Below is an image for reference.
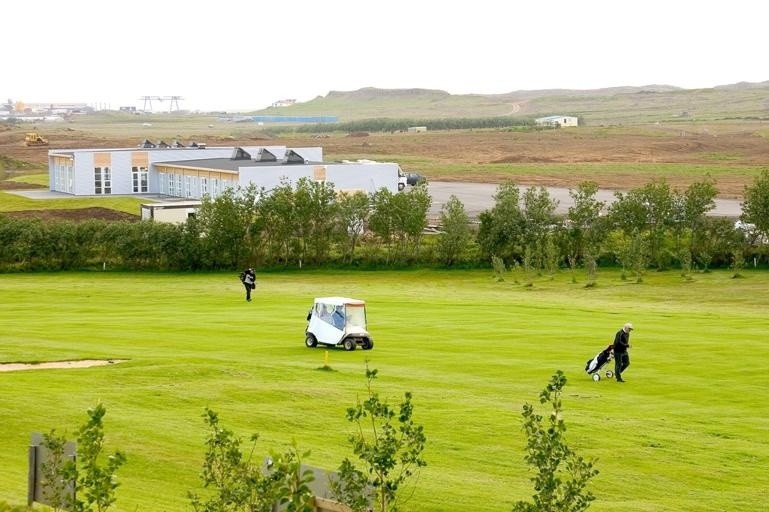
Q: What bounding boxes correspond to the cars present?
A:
[405,172,426,186]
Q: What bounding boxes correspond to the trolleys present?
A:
[585,345,614,382]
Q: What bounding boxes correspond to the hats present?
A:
[252,268,256,272]
[625,324,634,330]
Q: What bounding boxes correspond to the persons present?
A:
[320,305,332,316]
[239,263,256,302]
[613,323,634,383]
[333,305,353,332]
